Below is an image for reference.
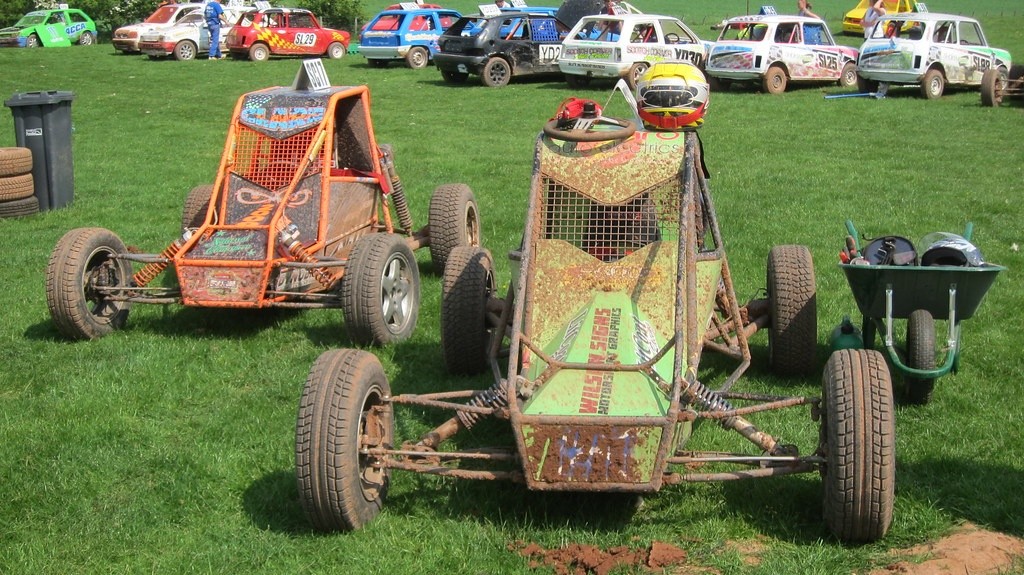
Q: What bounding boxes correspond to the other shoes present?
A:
[218,54,226,60]
[208,56,217,60]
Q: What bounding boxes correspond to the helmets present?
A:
[920,238,986,268]
[635,61,710,133]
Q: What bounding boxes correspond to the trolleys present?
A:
[835,218,1009,397]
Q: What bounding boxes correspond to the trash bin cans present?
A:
[4,88,75,211]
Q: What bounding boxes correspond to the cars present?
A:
[431,3,584,88]
[111,0,279,61]
[556,12,716,93]
[224,0,352,64]
[356,2,476,70]
[704,6,860,96]
[358,0,455,41]
[469,0,621,41]
[855,1,1012,101]
[0,4,99,49]
[841,0,922,35]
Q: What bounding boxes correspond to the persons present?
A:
[598,0,622,34]
[495,0,510,8]
[798,0,820,19]
[860,0,886,41]
[204,0,230,59]
